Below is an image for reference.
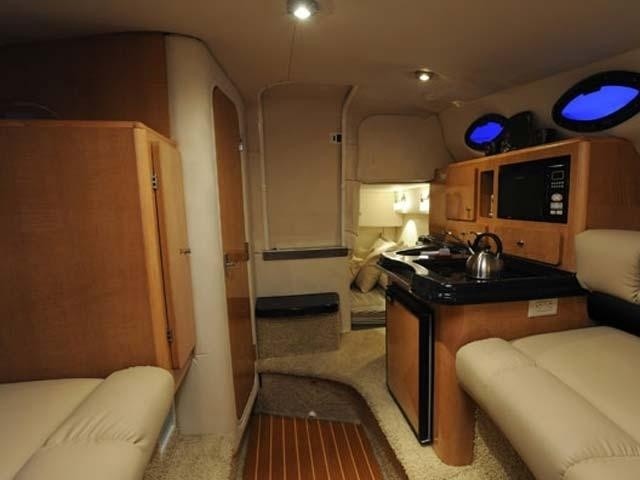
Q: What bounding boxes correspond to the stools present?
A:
[255,291,343,360]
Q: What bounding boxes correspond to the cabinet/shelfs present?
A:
[0,118,198,396]
[384,272,596,467]
[427,135,640,269]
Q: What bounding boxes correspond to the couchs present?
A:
[453,225,640,480]
[1,363,174,479]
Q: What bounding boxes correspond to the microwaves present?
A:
[496,154,572,223]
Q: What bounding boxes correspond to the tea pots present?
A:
[464,233,505,280]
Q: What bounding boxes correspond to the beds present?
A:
[349,280,385,324]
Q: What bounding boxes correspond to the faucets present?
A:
[443,229,463,245]
[468,230,481,245]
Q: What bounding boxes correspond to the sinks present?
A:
[395,244,463,257]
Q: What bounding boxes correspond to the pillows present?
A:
[378,271,389,289]
[355,233,398,294]
[349,256,366,283]
